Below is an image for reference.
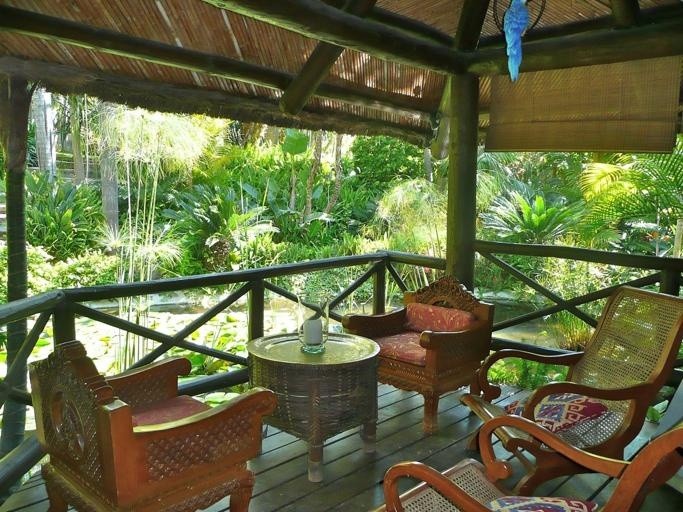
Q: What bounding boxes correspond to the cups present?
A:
[297,292,329,354]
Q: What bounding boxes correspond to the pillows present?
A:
[485,496,598,512]
[406,303,476,332]
[504,393,608,433]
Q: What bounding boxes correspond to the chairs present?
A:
[377,415,683,512]
[342,275,495,434]
[459,285,683,495]
[28,339,279,512]
